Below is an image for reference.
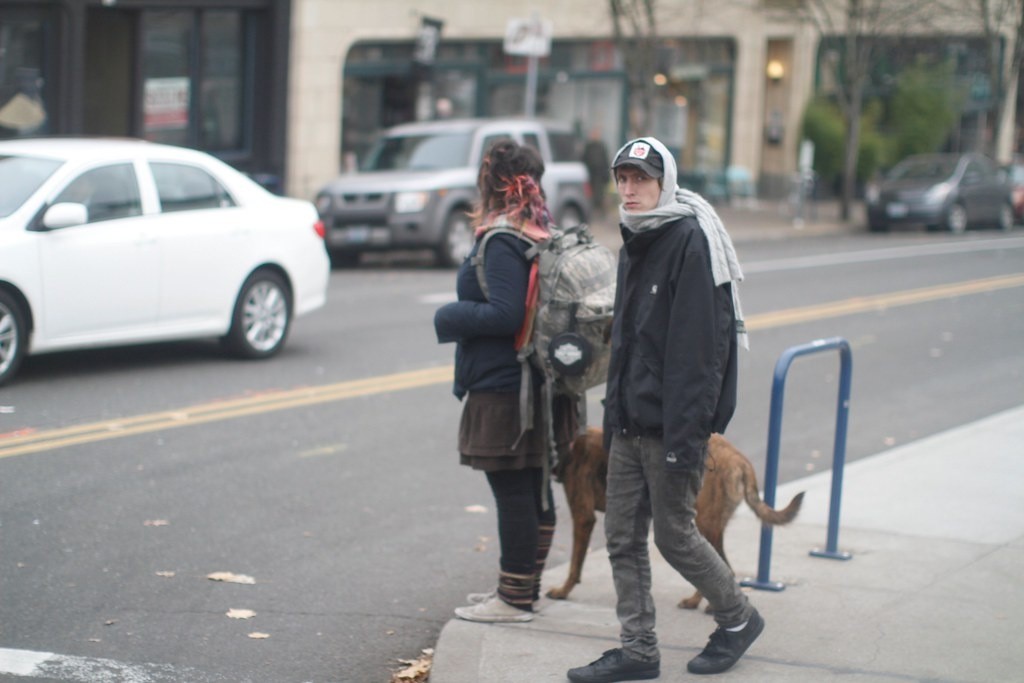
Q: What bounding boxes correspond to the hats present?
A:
[610,139,663,181]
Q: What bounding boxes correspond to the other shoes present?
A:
[455,599,535,621]
[466,590,498,604]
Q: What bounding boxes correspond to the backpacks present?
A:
[474,223,621,396]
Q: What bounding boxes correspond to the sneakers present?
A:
[687,609,764,674]
[566,646,659,682]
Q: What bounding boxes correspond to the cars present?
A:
[1002,160,1024,222]
[866,150,1013,229]
[0,137,330,382]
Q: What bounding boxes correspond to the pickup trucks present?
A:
[316,116,592,269]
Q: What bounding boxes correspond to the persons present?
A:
[558,137,769,682]
[433,139,585,623]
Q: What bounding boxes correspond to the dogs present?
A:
[547,424,806,615]
